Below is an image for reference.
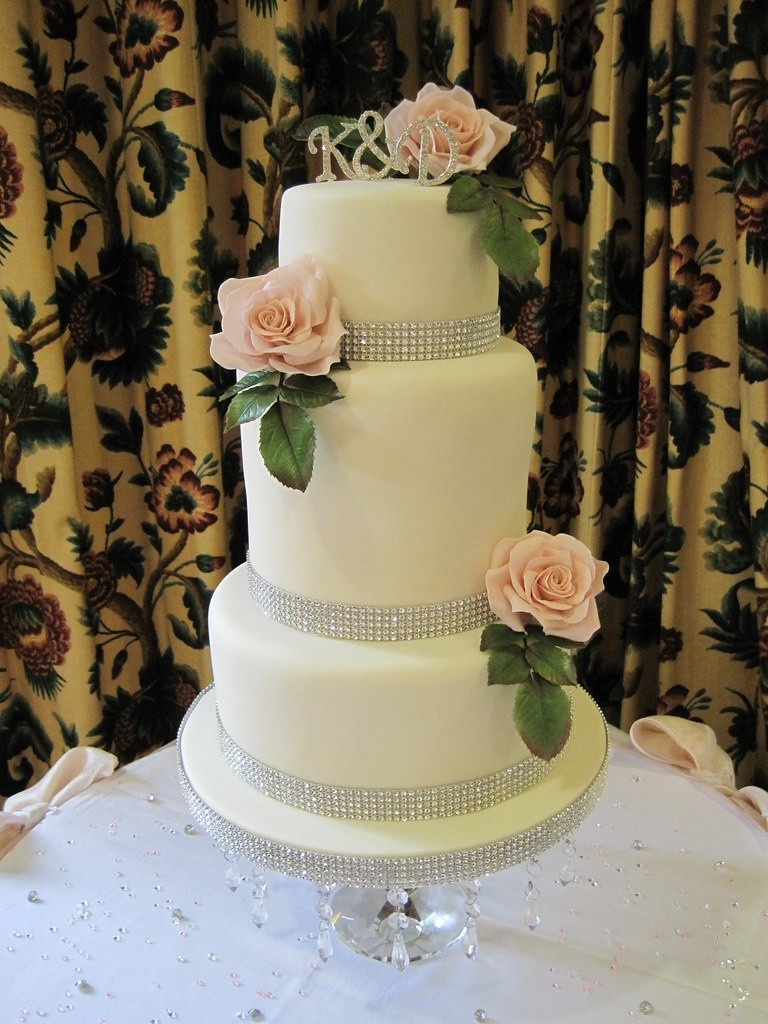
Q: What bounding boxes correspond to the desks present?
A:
[0,723,768,1024]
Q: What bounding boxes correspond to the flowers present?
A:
[201,250,351,496]
[293,81,540,293]
[479,530,610,766]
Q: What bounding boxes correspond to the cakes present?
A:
[174,81,611,887]
